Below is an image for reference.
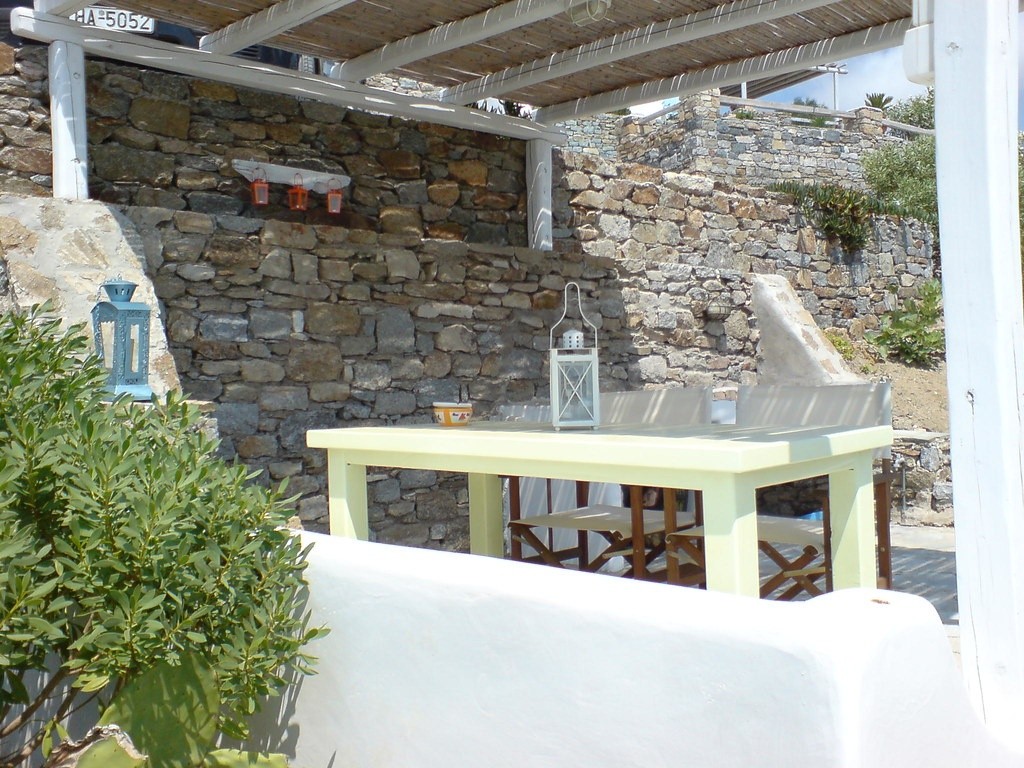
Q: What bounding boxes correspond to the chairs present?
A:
[662,382,895,601]
[497,385,713,588]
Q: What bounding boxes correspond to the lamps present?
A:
[89,272,154,403]
[700,298,733,322]
[546,280,602,431]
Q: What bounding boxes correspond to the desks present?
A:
[307,419,894,598]
